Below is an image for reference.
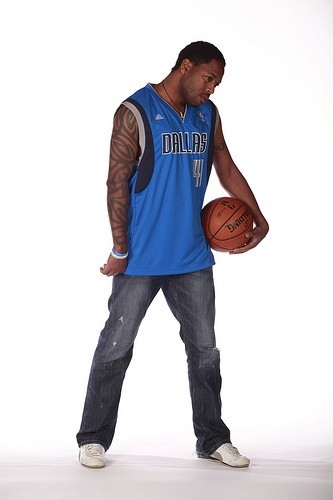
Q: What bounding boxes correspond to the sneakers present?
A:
[211,444,250,468]
[79,443,106,469]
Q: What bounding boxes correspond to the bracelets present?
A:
[111,248,128,259]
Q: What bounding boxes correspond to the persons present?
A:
[76,41,269,468]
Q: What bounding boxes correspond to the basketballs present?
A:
[201,197,253,252]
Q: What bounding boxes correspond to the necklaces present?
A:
[162,81,186,120]
